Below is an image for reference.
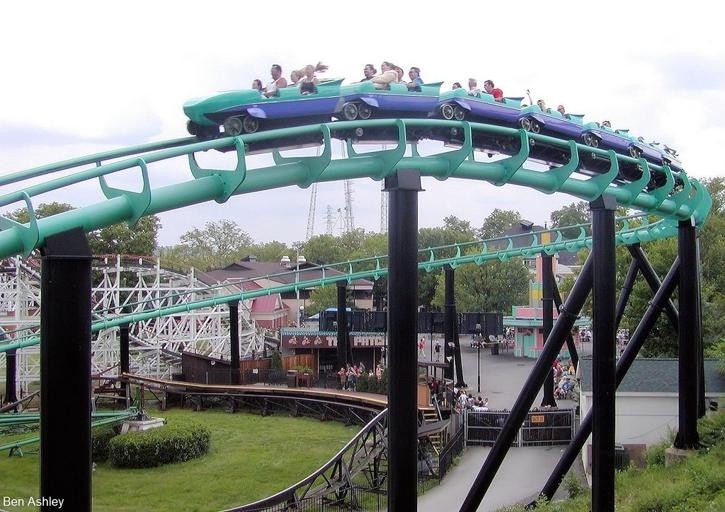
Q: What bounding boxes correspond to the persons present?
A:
[299,297,629,409]
[252,61,610,128]
[709,402,718,411]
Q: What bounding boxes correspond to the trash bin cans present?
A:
[287,370,298,387]
[491,343,498,355]
[615,444,629,470]
